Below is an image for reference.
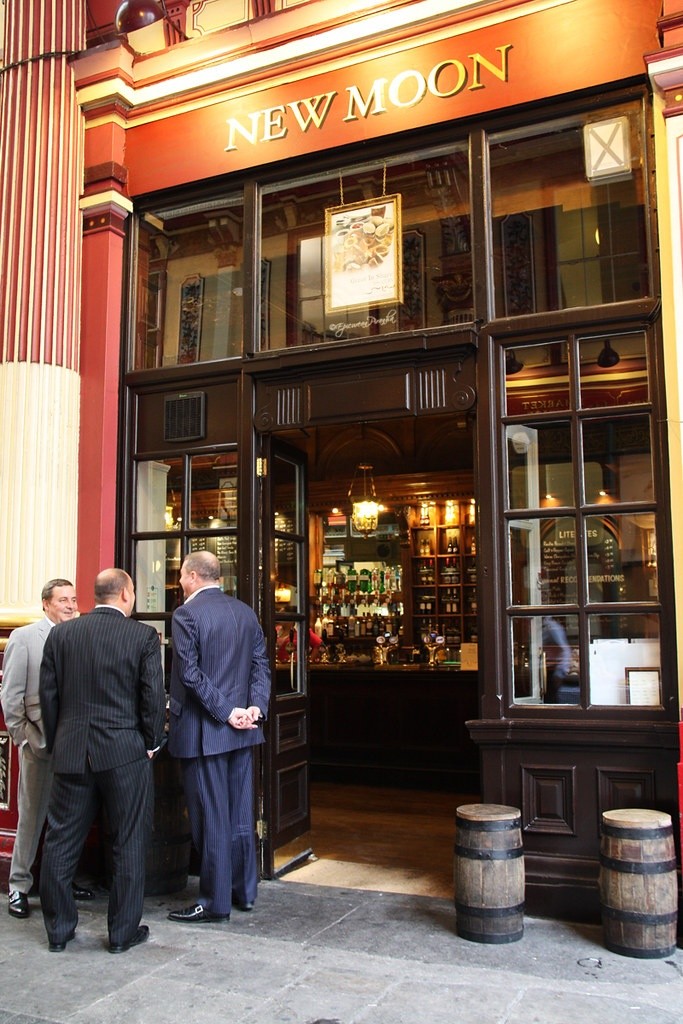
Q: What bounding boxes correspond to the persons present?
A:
[38,569,167,954]
[1,578,77,919]
[275,605,323,662]
[168,551,271,921]
[543,615,572,704]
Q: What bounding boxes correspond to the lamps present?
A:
[114,0,189,41]
[506,350,524,375]
[347,464,388,531]
[597,340,620,368]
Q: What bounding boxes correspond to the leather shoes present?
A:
[48,932,75,952]
[71,883,95,901]
[109,925,148,953]
[167,904,230,922]
[8,892,30,918]
[232,888,254,911]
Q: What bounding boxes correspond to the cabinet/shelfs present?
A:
[277,493,478,650]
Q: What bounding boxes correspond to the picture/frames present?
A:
[261,258,270,350]
[176,273,204,365]
[324,193,403,316]
[500,212,537,316]
[395,229,427,332]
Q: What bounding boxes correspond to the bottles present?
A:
[446,588,458,613]
[419,538,431,556]
[314,565,402,595]
[445,618,460,644]
[471,588,477,614]
[420,508,430,526]
[420,559,434,585]
[470,558,477,583]
[314,612,406,636]
[470,616,478,643]
[316,595,404,616]
[419,589,432,614]
[420,618,433,643]
[471,536,476,554]
[442,557,459,583]
[447,536,458,554]
